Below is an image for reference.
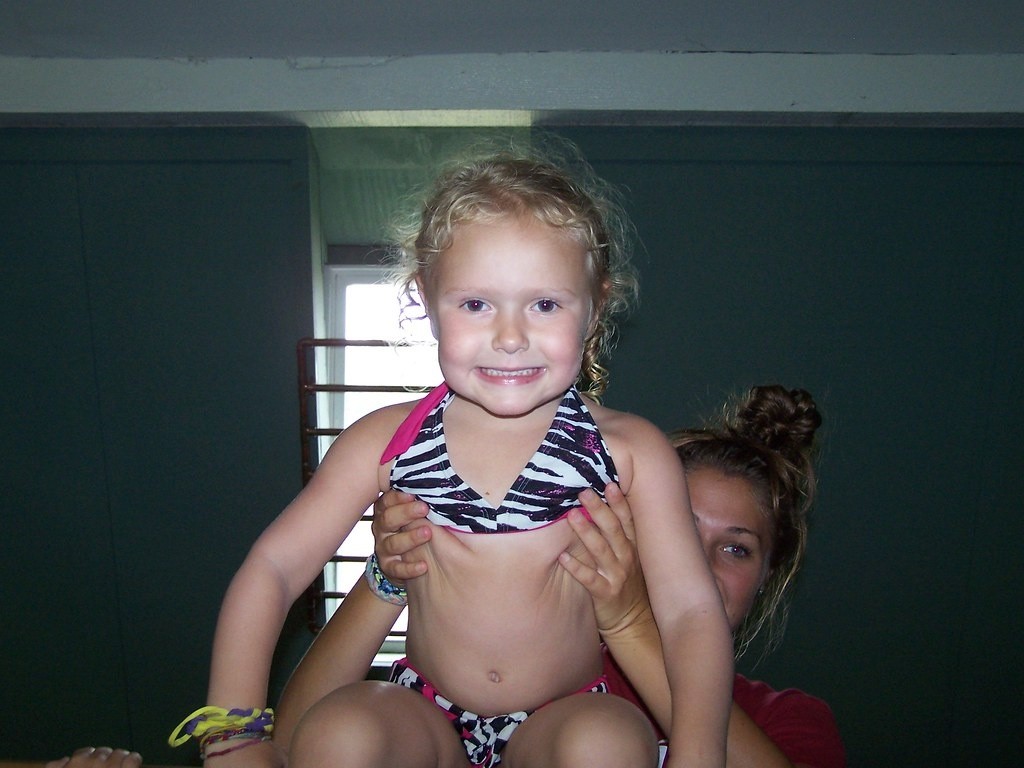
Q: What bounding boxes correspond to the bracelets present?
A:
[365,553,407,597]
[168,706,276,760]
[365,553,408,606]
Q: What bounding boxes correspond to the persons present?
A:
[272,385,847,768]
[201,150,732,768]
